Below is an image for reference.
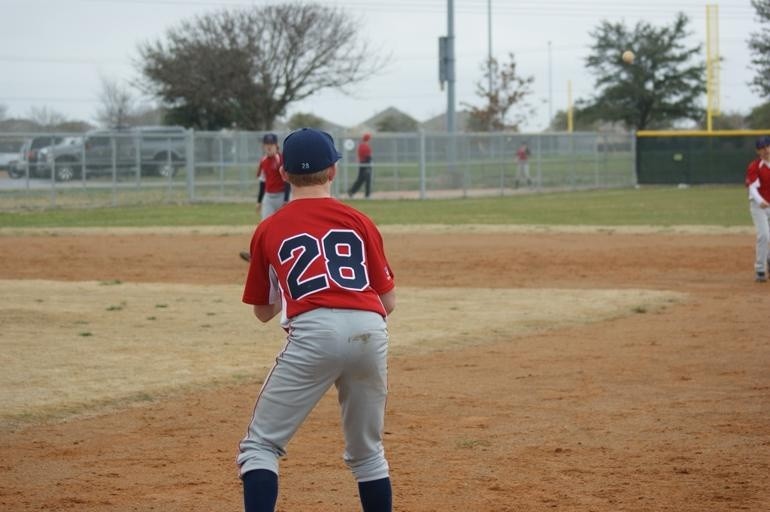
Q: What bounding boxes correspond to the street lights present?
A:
[623,51,646,130]
[547,40,553,123]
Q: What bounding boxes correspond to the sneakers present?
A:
[238,250,251,263]
[755,263,770,284]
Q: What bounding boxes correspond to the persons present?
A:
[236,128,396,511]
[347,133,372,199]
[515,143,533,188]
[744,136,770,282]
[256,133,291,221]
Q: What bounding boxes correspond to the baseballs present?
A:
[623,51,636,62]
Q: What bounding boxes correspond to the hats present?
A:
[282,127,343,177]
[262,133,279,146]
[755,136,770,149]
[362,132,372,142]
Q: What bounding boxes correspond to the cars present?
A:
[0,124,192,182]
